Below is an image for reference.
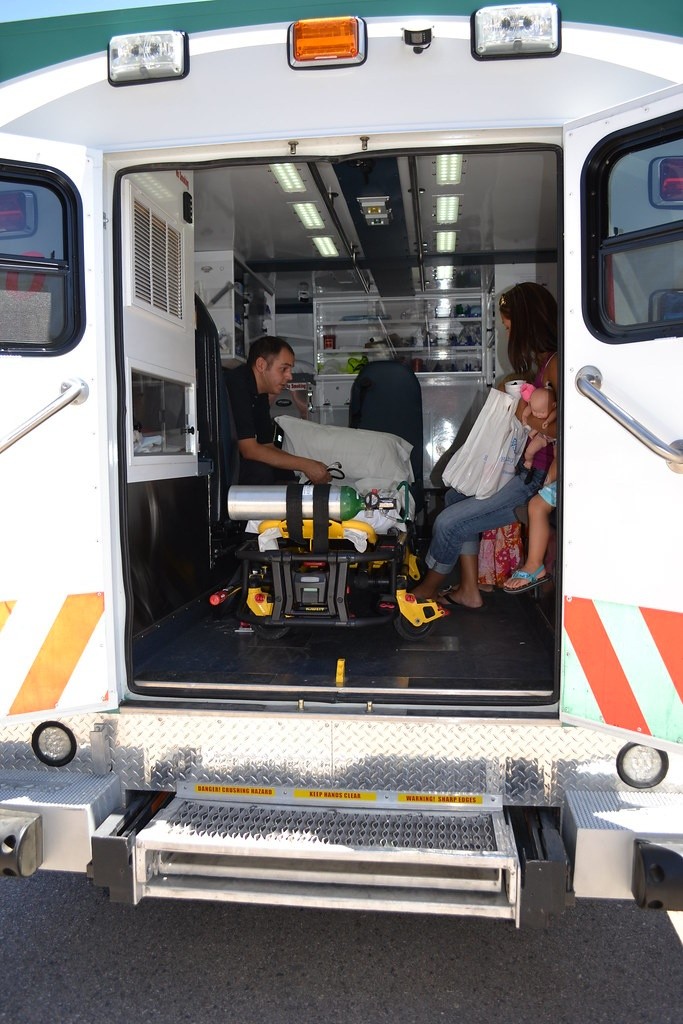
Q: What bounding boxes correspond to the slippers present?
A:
[436,591,489,614]
[478,583,495,594]
[438,585,457,597]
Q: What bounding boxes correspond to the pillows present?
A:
[275,415,415,485]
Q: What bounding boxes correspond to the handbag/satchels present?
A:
[441,387,529,501]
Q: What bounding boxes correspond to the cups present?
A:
[505,379,526,399]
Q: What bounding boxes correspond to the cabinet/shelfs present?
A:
[312,296,492,381]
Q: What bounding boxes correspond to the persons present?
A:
[220,335,333,487]
[521,388,558,469]
[502,460,556,594]
[410,282,558,610]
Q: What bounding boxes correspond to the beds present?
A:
[241,475,415,638]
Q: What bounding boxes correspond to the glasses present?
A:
[498,297,509,307]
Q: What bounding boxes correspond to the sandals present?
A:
[503,564,553,594]
